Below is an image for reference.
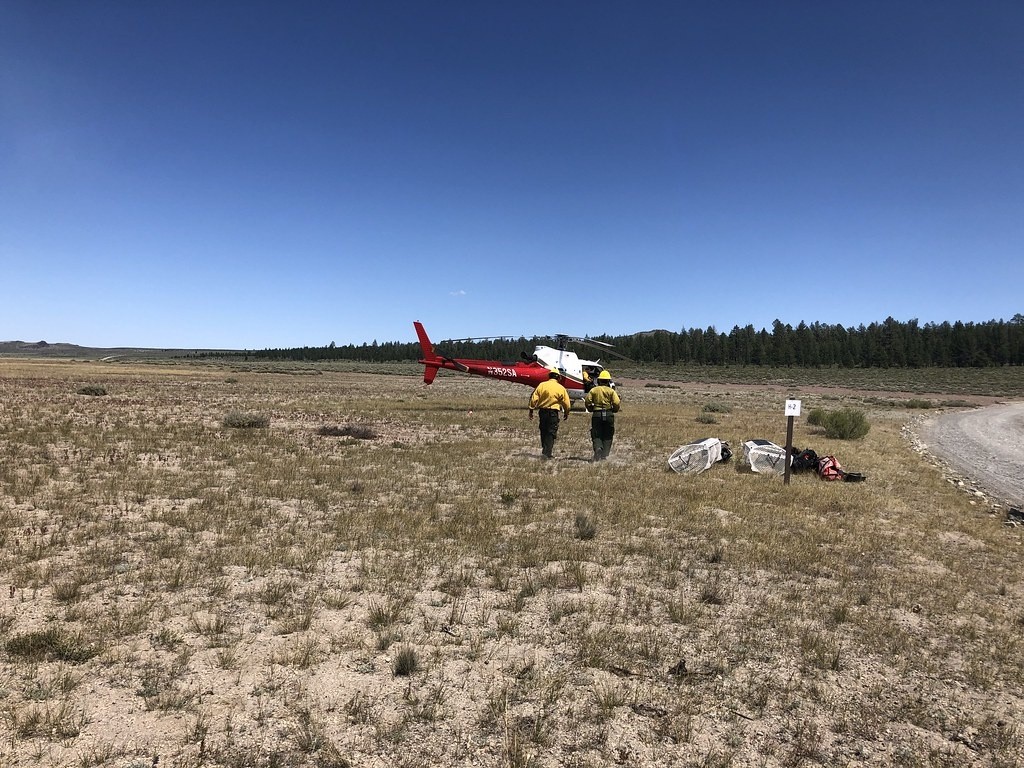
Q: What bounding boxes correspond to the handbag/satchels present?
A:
[781,446,866,481]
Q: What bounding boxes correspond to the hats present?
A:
[585,367,591,373]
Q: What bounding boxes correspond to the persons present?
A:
[528,369,571,458]
[583,368,593,386]
[585,370,620,459]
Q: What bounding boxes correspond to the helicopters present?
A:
[412,320,617,414]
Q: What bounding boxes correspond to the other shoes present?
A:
[595,448,602,460]
[601,456,607,460]
[542,452,555,459]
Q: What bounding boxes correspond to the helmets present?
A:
[548,369,561,376]
[598,370,612,380]
